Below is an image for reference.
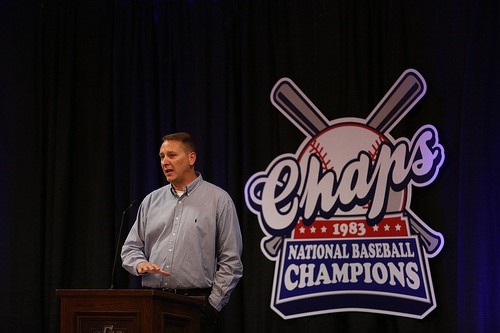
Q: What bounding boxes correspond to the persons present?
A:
[120,130,244,332]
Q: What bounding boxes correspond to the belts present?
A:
[156,285,214,297]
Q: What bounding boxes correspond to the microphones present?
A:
[110,201,134,287]
